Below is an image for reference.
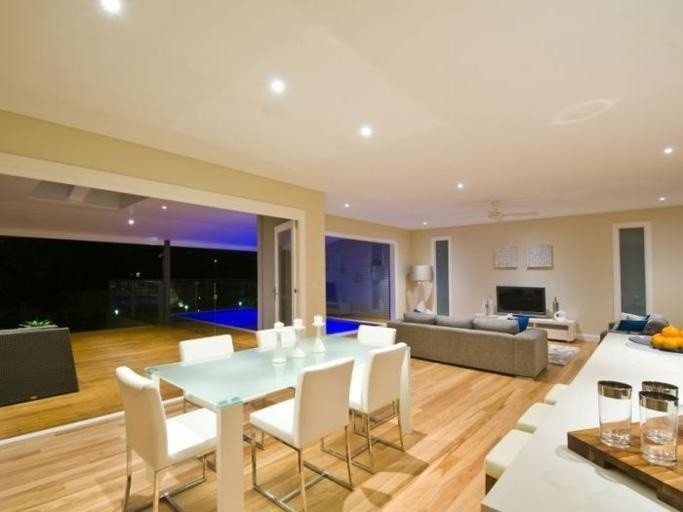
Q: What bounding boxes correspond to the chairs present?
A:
[254,325,298,346]
[320,340,410,477]
[355,323,396,348]
[595,311,671,345]
[114,366,221,512]
[178,331,267,474]
[247,356,355,512]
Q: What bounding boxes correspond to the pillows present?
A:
[616,319,648,332]
[507,313,529,333]
[610,311,649,330]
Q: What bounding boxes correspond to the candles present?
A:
[313,315,322,325]
[294,318,303,328]
[274,320,285,329]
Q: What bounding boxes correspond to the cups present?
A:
[642,377,679,403]
[597,378,633,449]
[638,391,679,467]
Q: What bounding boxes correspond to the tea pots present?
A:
[554,310,568,322]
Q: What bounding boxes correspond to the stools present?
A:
[515,400,555,431]
[540,379,571,406]
[475,425,533,493]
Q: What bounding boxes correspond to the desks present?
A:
[136,329,413,512]
[470,327,682,511]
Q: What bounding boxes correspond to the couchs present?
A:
[385,310,550,379]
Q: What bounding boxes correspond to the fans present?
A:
[486,195,538,220]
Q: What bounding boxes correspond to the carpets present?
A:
[546,341,580,367]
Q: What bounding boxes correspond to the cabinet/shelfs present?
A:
[473,312,578,344]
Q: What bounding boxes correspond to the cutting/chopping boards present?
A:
[566,413,683,510]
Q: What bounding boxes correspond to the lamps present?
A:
[407,262,433,313]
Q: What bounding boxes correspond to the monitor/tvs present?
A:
[496,286,546,320]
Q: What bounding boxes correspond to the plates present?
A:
[629,335,650,347]
